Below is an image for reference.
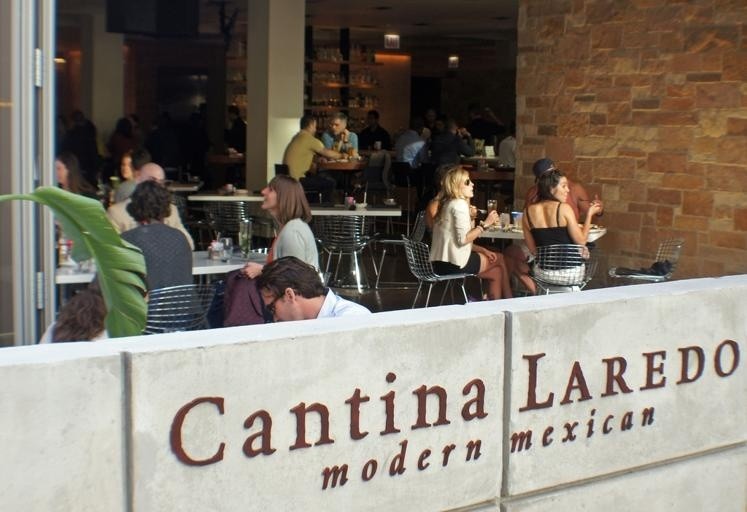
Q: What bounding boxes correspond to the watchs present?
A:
[342,154,344,158]
[344,142,349,143]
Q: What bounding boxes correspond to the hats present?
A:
[533,158,553,183]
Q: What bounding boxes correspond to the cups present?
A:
[220,238,233,263]
[238,220,252,259]
[512,212,523,227]
[487,199,498,216]
[500,213,510,232]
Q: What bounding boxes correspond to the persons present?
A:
[431,122,475,186]
[225,105,247,153]
[107,162,195,250]
[498,126,515,211]
[105,118,142,181]
[421,165,477,252]
[388,119,432,213]
[284,116,348,207]
[521,159,604,222]
[224,174,319,327]
[57,113,83,161]
[505,169,602,295]
[56,150,95,197]
[89,180,202,333]
[136,111,182,181]
[319,113,362,202]
[121,148,136,180]
[424,108,437,130]
[71,109,99,191]
[116,151,151,203]
[430,115,448,138]
[128,113,149,157]
[484,105,507,135]
[358,110,391,150]
[429,167,513,300]
[256,257,372,322]
[466,105,505,150]
[39,270,149,343]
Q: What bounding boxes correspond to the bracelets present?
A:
[477,220,488,232]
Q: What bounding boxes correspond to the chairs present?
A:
[202,200,254,240]
[375,210,427,290]
[312,215,379,295]
[273,163,323,208]
[401,232,484,309]
[143,284,217,336]
[386,161,417,238]
[528,243,599,295]
[609,237,688,282]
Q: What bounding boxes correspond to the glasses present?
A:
[551,169,560,184]
[328,123,340,127]
[464,178,470,186]
[266,288,299,314]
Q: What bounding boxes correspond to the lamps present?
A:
[54,51,68,67]
